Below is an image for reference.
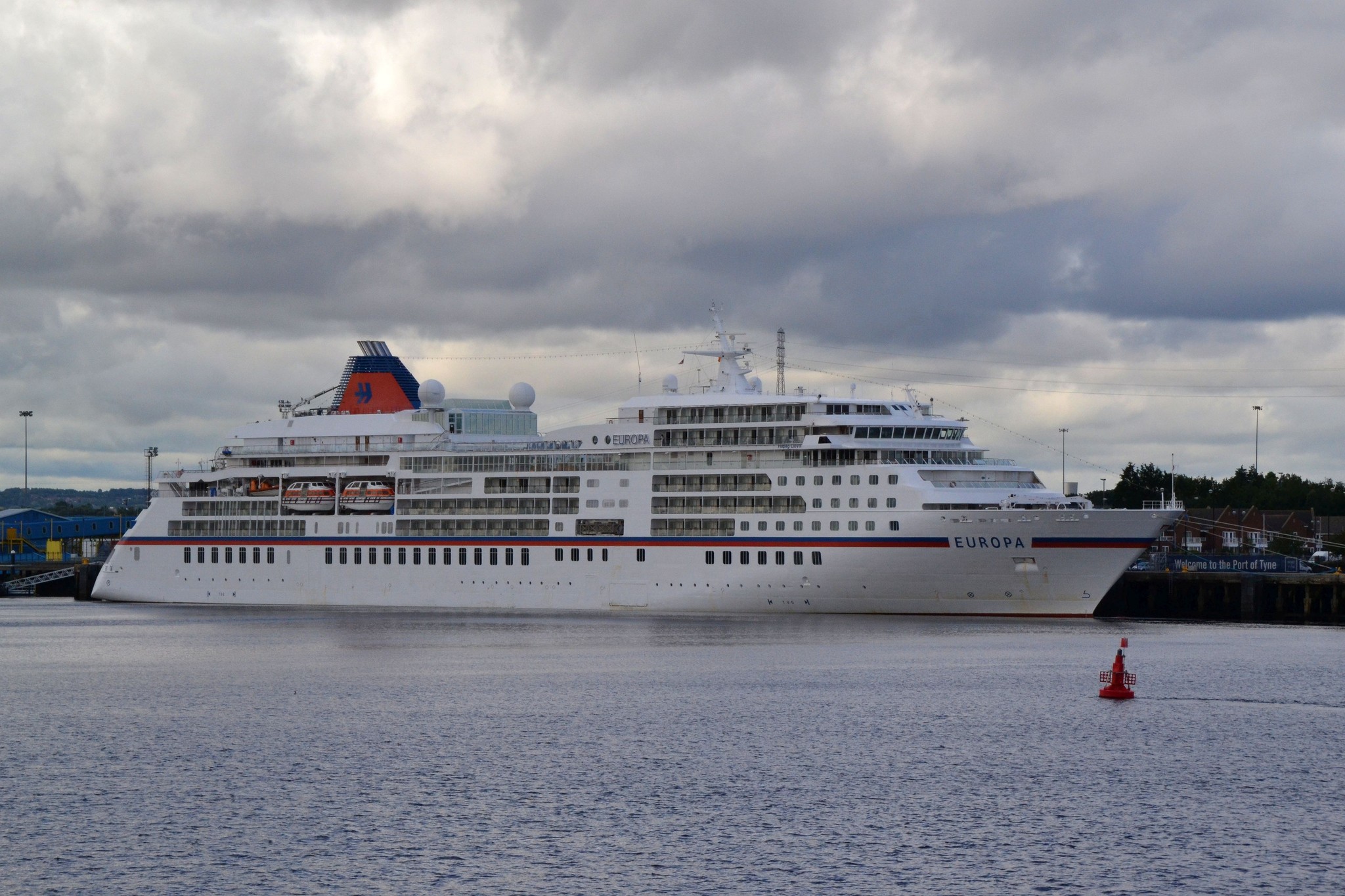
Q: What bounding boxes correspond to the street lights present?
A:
[1252,406,1263,471]
[19,410,33,496]
[148,446,159,507]
[1194,489,1215,555]
[1311,516,1320,552]
[1100,478,1106,509]
[1059,429,1069,495]
[1232,510,1246,555]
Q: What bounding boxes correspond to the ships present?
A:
[88,298,1192,628]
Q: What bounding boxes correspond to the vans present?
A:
[1308,551,1343,563]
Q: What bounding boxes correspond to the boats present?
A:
[250,488,287,496]
[282,481,335,512]
[340,480,395,511]
